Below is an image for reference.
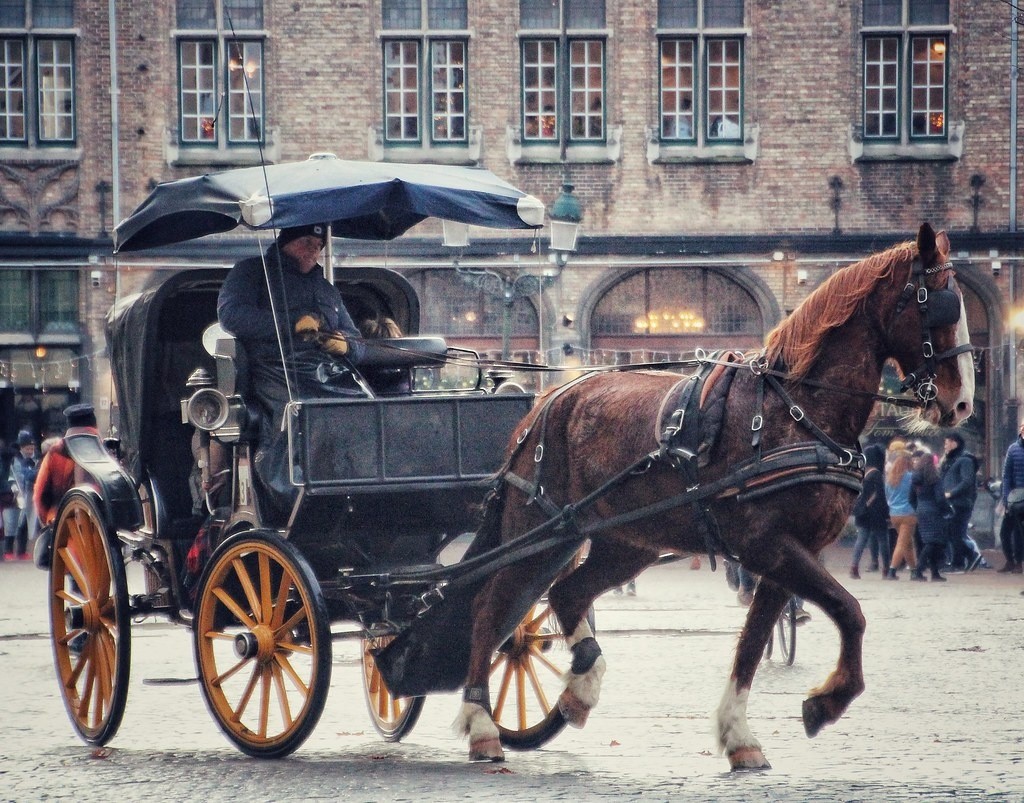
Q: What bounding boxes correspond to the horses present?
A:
[450,219,977,770]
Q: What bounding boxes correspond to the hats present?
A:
[278,224,327,251]
[10,431,35,445]
[62,403,97,427]
[968,523,974,529]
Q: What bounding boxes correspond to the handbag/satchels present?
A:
[1007,488,1024,513]
[16,493,26,509]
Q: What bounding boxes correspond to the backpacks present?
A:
[852,466,880,517]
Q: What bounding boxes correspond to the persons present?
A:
[722,419,1024,620]
[613,580,637,596]
[0,404,102,600]
[217,220,371,452]
[357,316,411,396]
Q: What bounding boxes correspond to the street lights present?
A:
[440,182,584,361]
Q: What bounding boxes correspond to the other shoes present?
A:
[888,568,897,578]
[2,554,15,561]
[931,569,946,582]
[850,564,861,579]
[1012,563,1022,573]
[882,569,899,580]
[16,553,33,561]
[983,562,993,568]
[911,568,917,579]
[866,563,879,572]
[966,552,983,572]
[915,563,927,581]
[997,560,1015,572]
[941,566,965,574]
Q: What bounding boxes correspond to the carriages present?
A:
[33,153,977,773]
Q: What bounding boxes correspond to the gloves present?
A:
[296,316,319,332]
[322,331,349,356]
